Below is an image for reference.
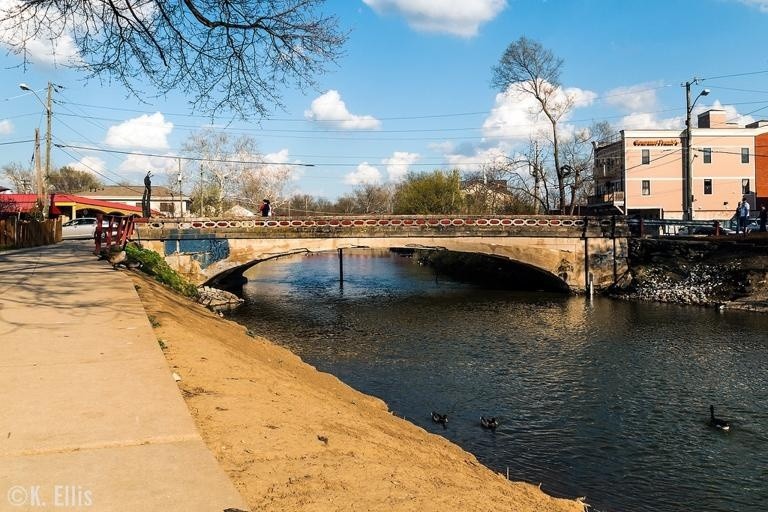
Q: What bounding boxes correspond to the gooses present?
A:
[708,404,731,430]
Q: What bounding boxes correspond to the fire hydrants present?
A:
[713,220,720,237]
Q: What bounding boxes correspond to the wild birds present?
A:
[97,250,127,269]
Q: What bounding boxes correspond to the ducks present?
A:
[123,260,144,271]
[431,411,448,424]
[480,415,499,428]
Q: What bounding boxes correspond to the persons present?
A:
[257,199,269,225]
[736,198,766,235]
[267,200,272,225]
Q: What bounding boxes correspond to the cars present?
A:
[679,225,734,236]
[61,216,120,240]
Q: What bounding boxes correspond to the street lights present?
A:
[683,86,711,220]
[19,81,53,218]
